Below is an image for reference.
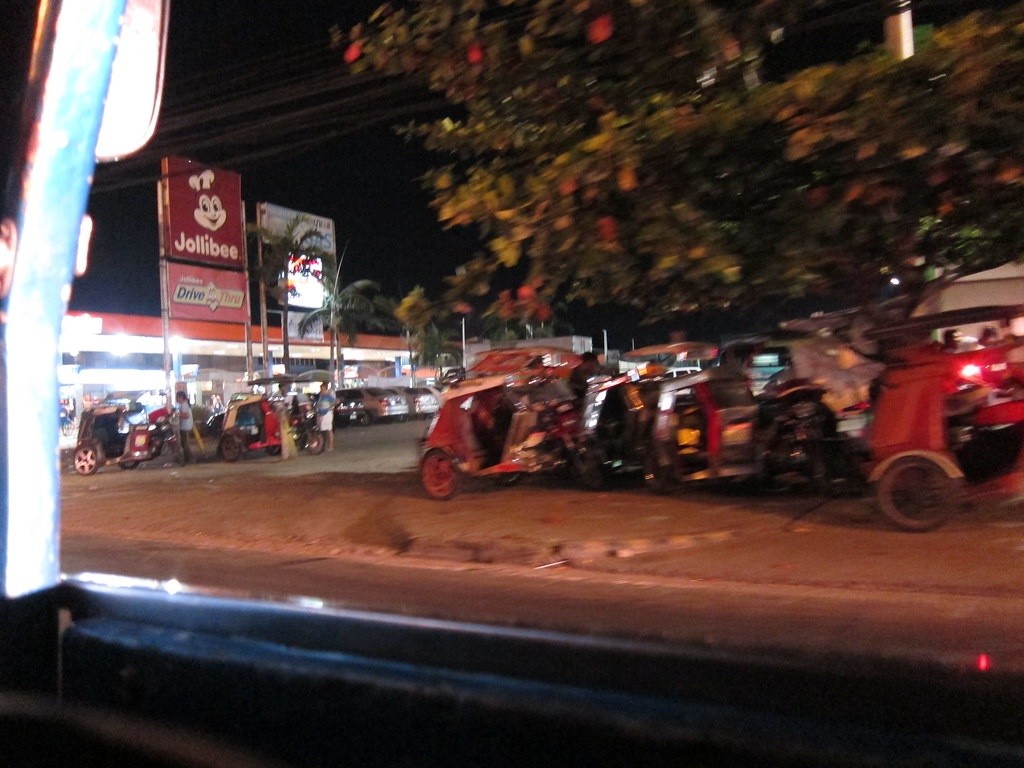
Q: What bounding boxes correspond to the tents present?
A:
[780,261,1024,380]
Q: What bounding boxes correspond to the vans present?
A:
[717,335,793,406]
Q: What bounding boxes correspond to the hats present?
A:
[581,352,596,360]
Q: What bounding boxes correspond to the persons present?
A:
[176,391,197,466]
[981,324,1004,343]
[943,329,965,353]
[317,383,339,450]
[472,385,520,470]
[568,351,611,407]
[58,403,68,436]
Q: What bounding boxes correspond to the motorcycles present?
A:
[72,392,186,477]
[217,394,326,461]
[579,344,761,496]
[752,304,1024,532]
[417,346,585,498]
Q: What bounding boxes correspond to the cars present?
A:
[398,386,440,422]
[358,387,409,427]
[206,392,316,433]
[311,392,366,428]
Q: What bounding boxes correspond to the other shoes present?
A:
[326,447,334,452]
